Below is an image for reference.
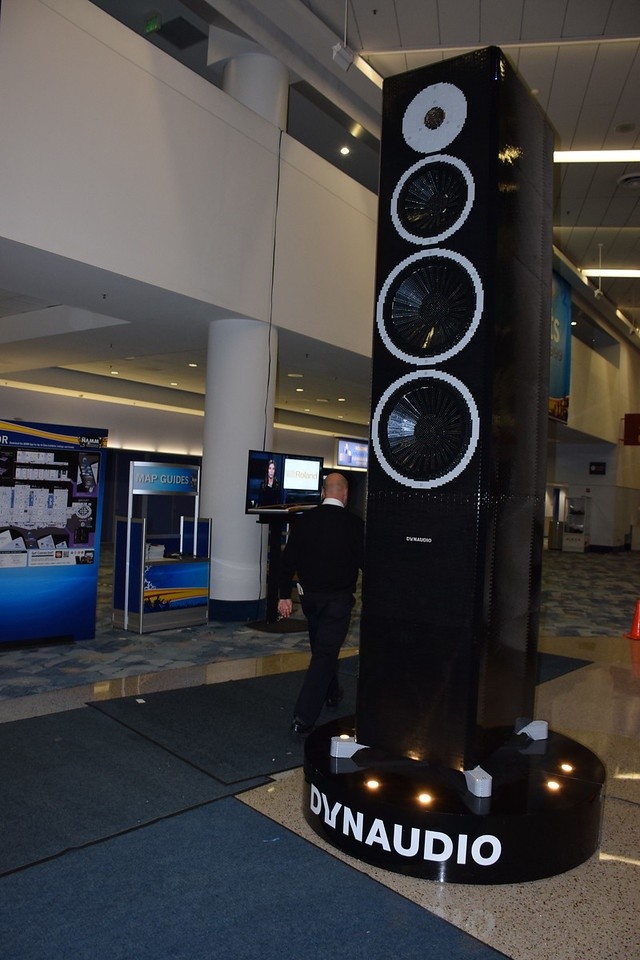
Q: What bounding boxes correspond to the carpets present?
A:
[0,651,596,960]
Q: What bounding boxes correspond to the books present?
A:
[145,543,165,560]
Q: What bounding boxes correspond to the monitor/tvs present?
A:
[338,441,368,469]
[243,449,325,515]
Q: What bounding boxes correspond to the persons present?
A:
[277,473,365,732]
[257,460,280,506]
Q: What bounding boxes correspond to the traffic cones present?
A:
[623,600,640,639]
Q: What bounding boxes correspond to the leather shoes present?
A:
[326,693,344,711]
[290,723,317,739]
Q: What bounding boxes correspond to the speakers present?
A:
[356,45,559,764]
